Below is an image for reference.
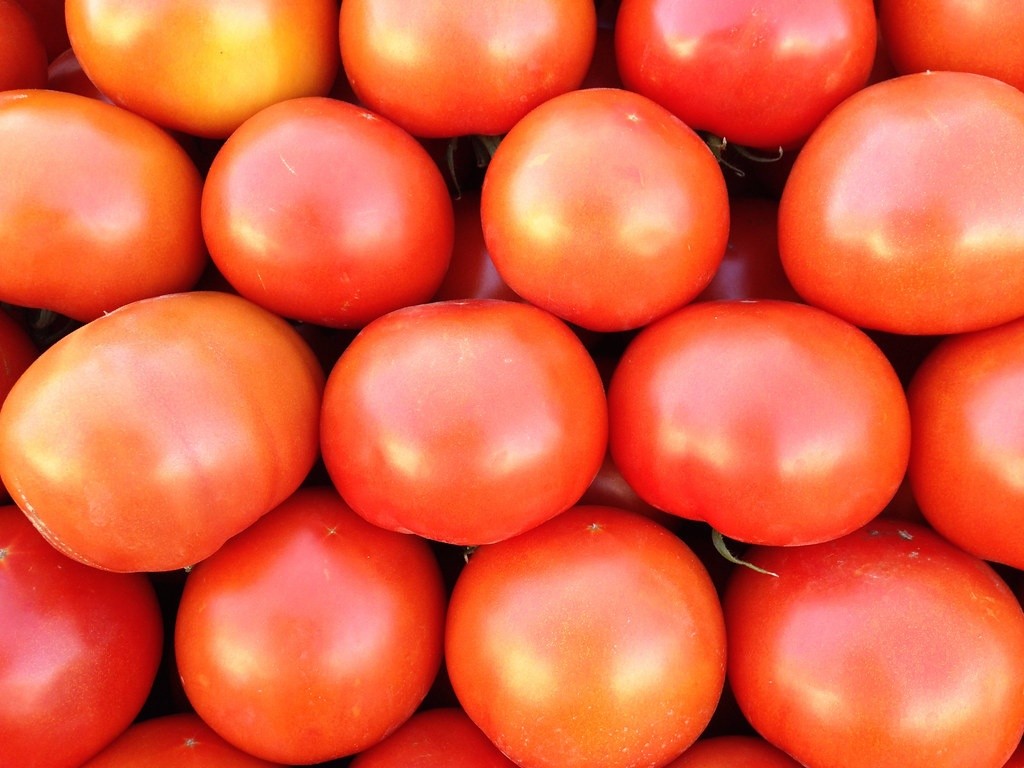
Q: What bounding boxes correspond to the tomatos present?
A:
[0,0,1024,768]
